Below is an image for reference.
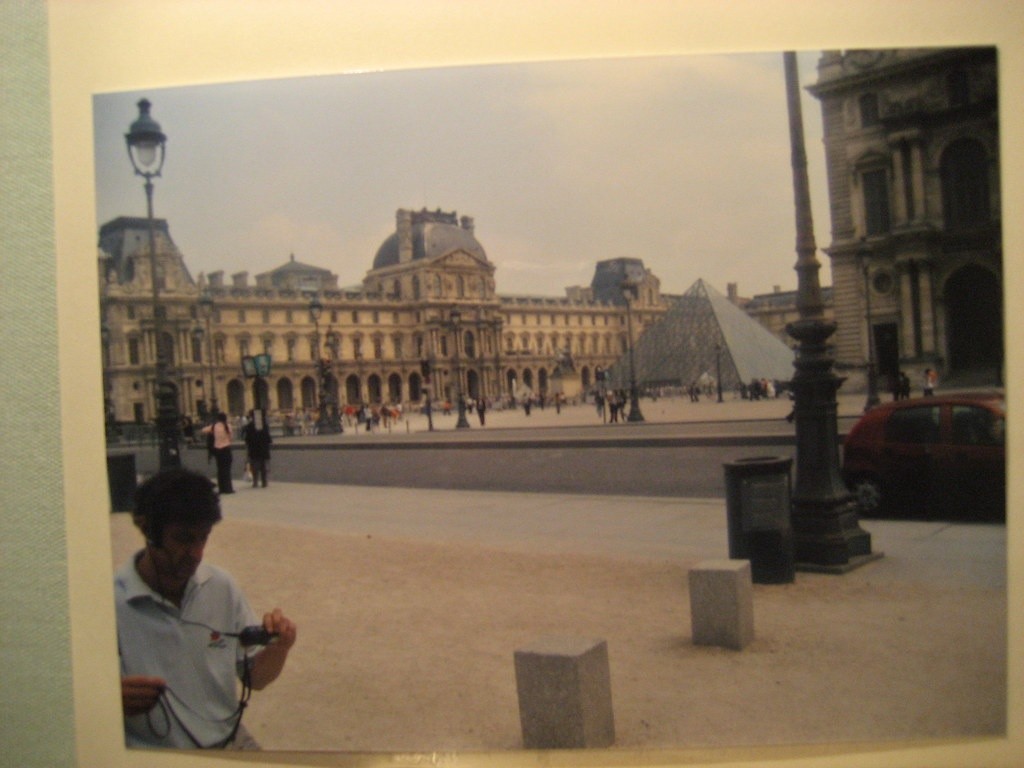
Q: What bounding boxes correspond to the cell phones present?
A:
[240,625,280,646]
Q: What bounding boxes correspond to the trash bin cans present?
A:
[107,454,138,512]
[723,456,795,584]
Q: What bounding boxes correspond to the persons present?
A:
[923,368,936,397]
[892,372,910,400]
[114,464,296,750]
[202,413,236,494]
[984,418,1003,445]
[245,409,271,487]
[237,378,790,440]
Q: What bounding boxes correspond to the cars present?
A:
[842,398,1005,522]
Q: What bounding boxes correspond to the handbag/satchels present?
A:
[206,424,215,463]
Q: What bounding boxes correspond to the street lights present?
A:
[713,341,724,403]
[450,304,471,428]
[852,236,882,412]
[124,97,182,472]
[618,274,644,421]
[308,296,333,434]
[199,287,220,423]
